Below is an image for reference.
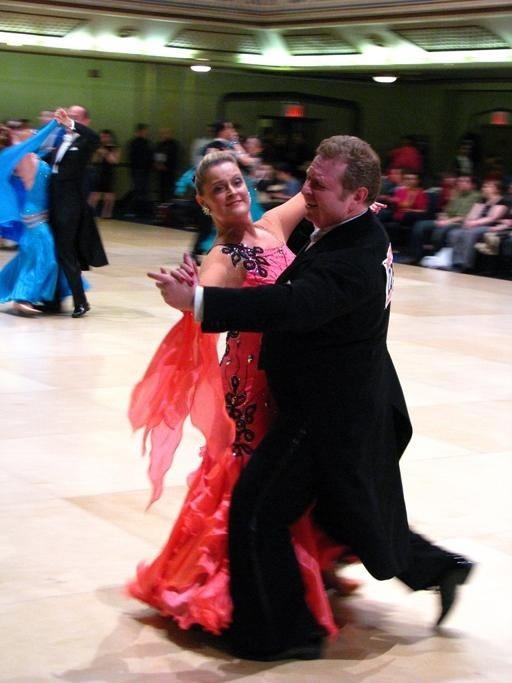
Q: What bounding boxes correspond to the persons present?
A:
[89,119,313,265]
[144,132,474,663]
[35,105,109,318]
[372,139,511,272]
[124,143,366,643]
[0,110,60,317]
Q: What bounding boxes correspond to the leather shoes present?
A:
[72,302,90,318]
[436,554,472,624]
